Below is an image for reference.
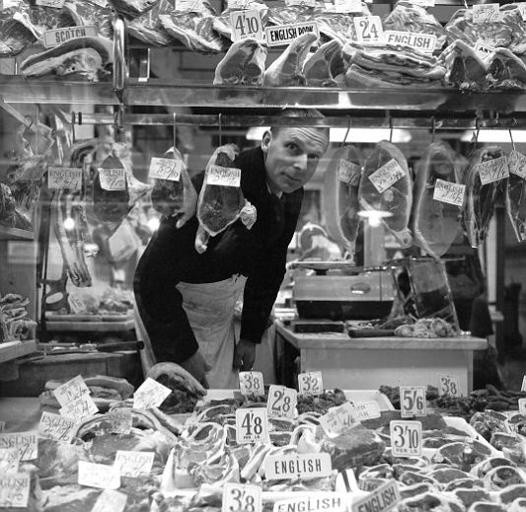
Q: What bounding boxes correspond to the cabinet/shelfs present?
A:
[1,99,39,363]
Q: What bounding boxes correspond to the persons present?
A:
[130,107,330,390]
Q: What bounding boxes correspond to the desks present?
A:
[273,318,487,399]
